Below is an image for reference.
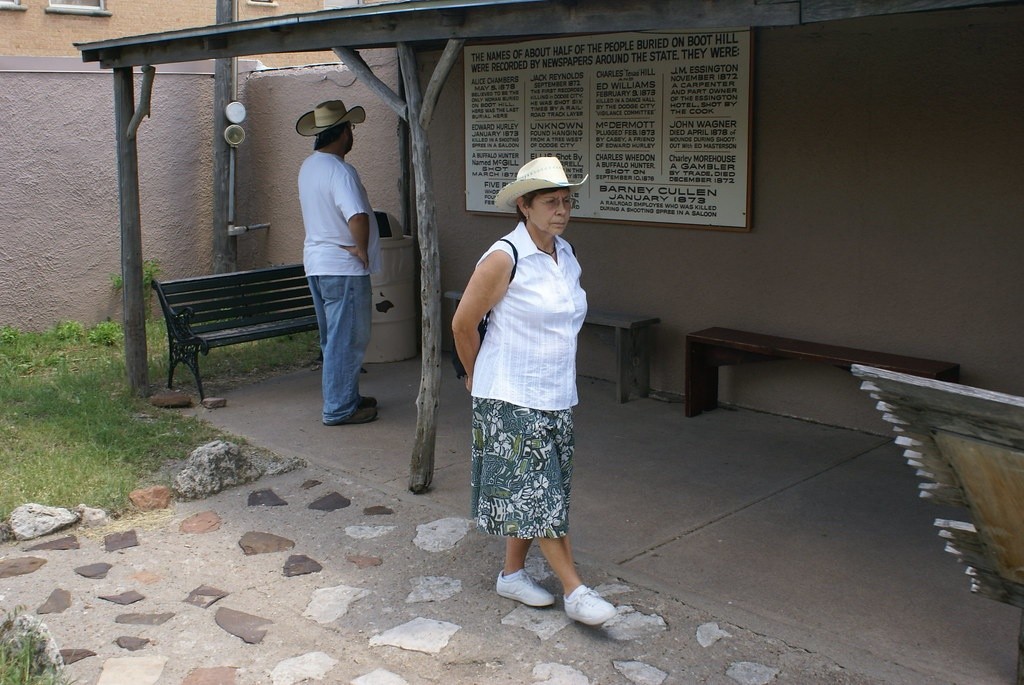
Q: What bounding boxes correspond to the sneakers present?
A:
[496,569,555,607]
[339,408,378,423]
[563,584,618,626]
[358,396,377,409]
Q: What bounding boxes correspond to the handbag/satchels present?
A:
[450,310,492,380]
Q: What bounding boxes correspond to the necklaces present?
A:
[536,245,557,257]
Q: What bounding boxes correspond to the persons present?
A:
[295,100,381,424]
[451,155,616,625]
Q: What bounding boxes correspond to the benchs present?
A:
[152,264,368,402]
[444,289,661,404]
[851,364,1024,685]
[686,327,960,421]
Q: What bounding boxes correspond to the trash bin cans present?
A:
[357,210,419,363]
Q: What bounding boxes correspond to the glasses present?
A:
[339,124,355,133]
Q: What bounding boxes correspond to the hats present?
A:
[492,156,590,213]
[295,100,365,137]
